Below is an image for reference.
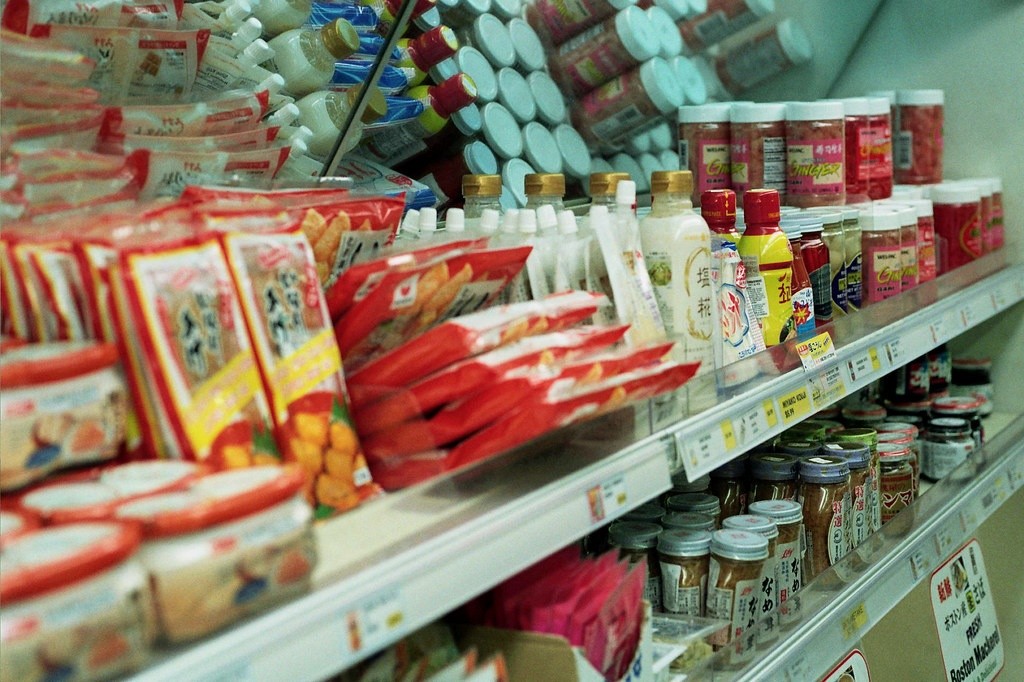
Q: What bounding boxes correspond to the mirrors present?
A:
[0,0,886,223]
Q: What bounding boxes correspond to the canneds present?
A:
[676,85,1006,366]
[411,0,813,210]
[588,345,995,672]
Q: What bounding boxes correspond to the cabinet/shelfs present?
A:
[117,261,1024,682]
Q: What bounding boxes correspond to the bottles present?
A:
[394,88,1006,415]
[599,421,879,669]
[217,1,813,212]
